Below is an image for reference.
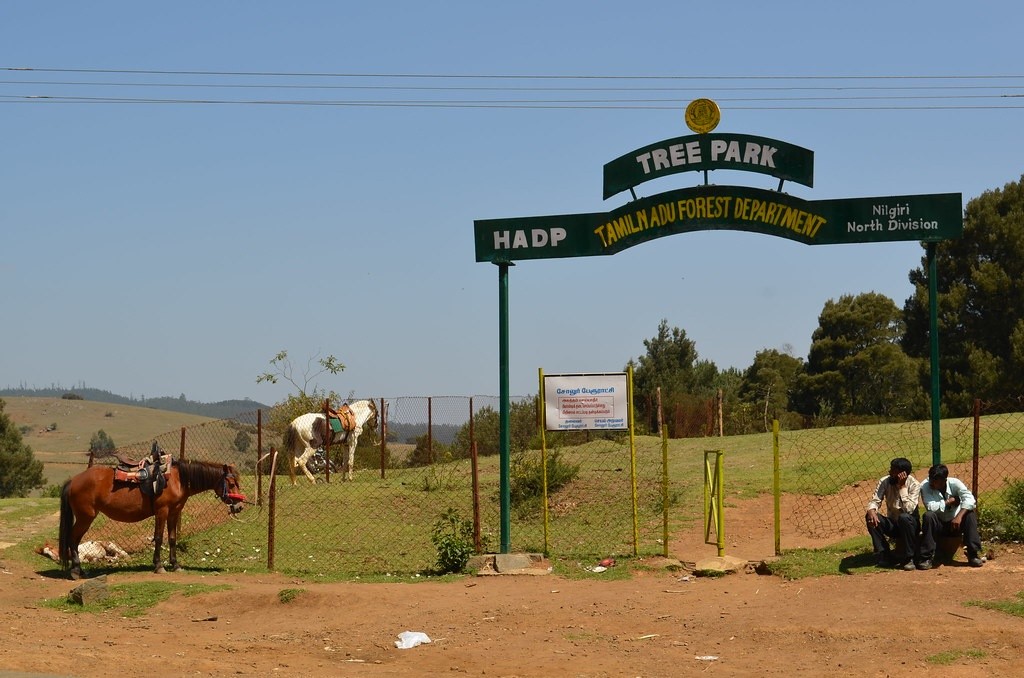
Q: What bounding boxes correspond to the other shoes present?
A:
[903,557,915,570]
[878,548,891,567]
[916,554,933,569]
[967,550,982,567]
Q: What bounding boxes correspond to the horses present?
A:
[283,400,379,487]
[34,539,129,565]
[56,442,244,580]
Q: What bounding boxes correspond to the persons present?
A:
[864,456,922,570]
[918,464,986,569]
